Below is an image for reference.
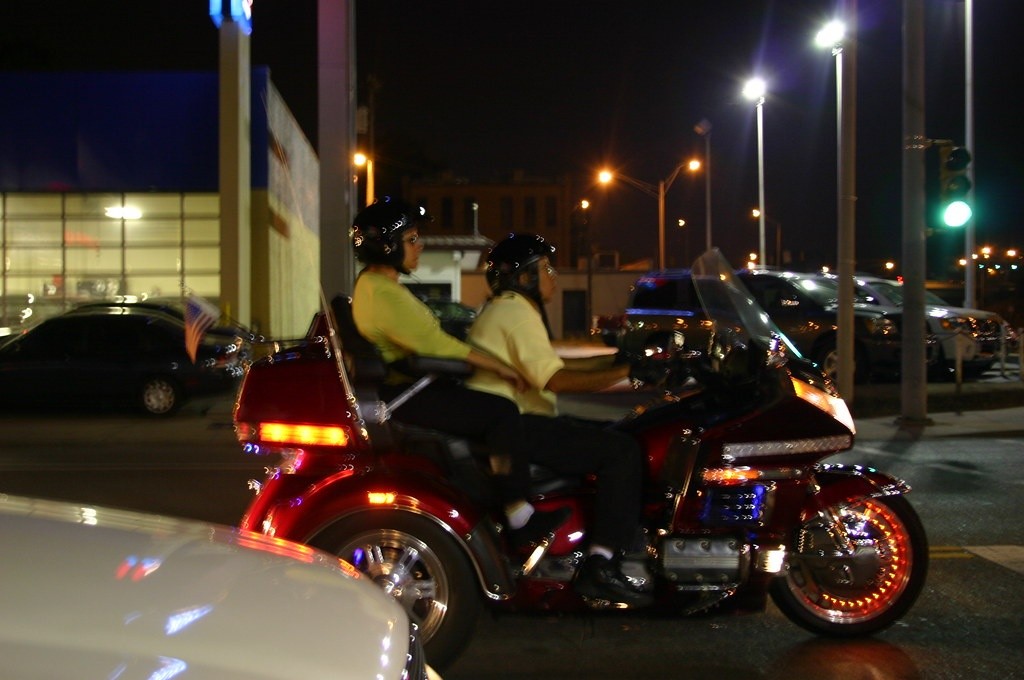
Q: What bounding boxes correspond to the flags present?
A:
[185,294,221,362]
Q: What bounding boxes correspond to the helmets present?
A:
[486,232,556,295]
[352,197,435,265]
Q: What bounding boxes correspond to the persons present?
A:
[351,202,575,549]
[465,231,646,603]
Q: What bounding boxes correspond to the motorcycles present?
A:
[233,244,934,641]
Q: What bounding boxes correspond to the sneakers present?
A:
[507,505,572,549]
[578,554,649,605]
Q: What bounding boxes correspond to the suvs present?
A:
[601,266,1008,404]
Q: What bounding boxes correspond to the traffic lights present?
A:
[935,145,973,229]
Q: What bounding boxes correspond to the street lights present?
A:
[811,18,855,406]
[596,157,703,272]
[742,77,767,273]
[695,116,713,250]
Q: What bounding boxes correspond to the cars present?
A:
[2,491,449,679]
[0,305,251,413]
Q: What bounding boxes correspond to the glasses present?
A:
[401,234,418,246]
